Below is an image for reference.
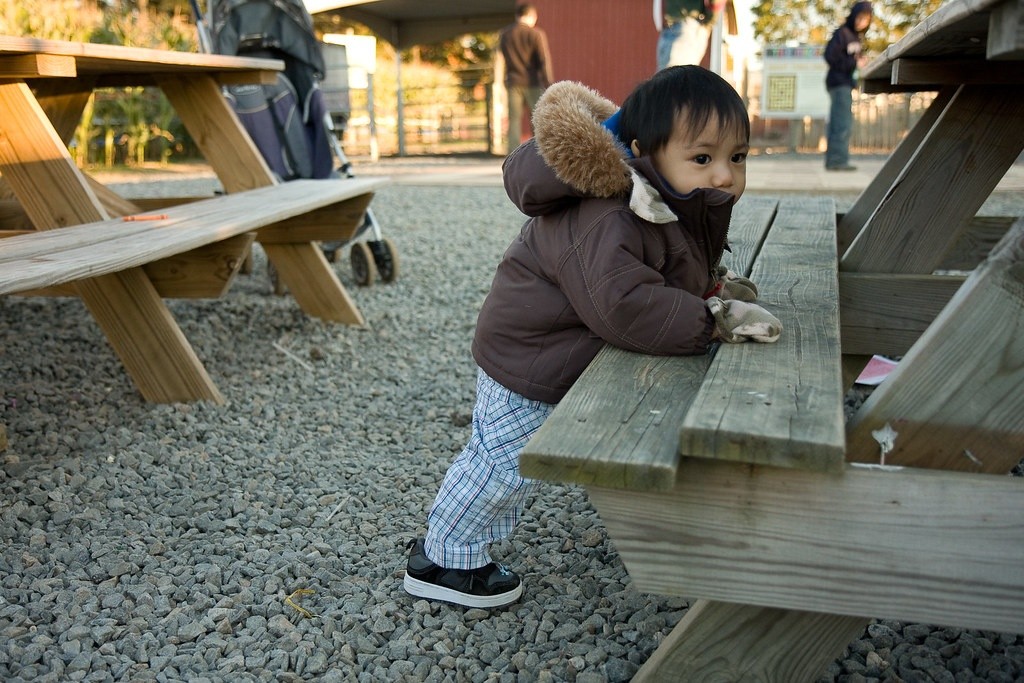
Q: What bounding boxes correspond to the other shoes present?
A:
[826,165,857,172]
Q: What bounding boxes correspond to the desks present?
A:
[627,0,1024,683]
[0,36,365,405]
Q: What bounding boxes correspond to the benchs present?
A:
[519,189,844,490]
[0,175,393,297]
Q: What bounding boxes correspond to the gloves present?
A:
[712,266,758,300]
[706,296,783,343]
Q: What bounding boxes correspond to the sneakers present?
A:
[403,538,523,607]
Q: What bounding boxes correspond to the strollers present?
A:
[198,0,400,288]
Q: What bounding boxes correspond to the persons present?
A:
[499,4,556,157]
[820,3,874,169]
[404,62,785,610]
[653,0,726,72]
